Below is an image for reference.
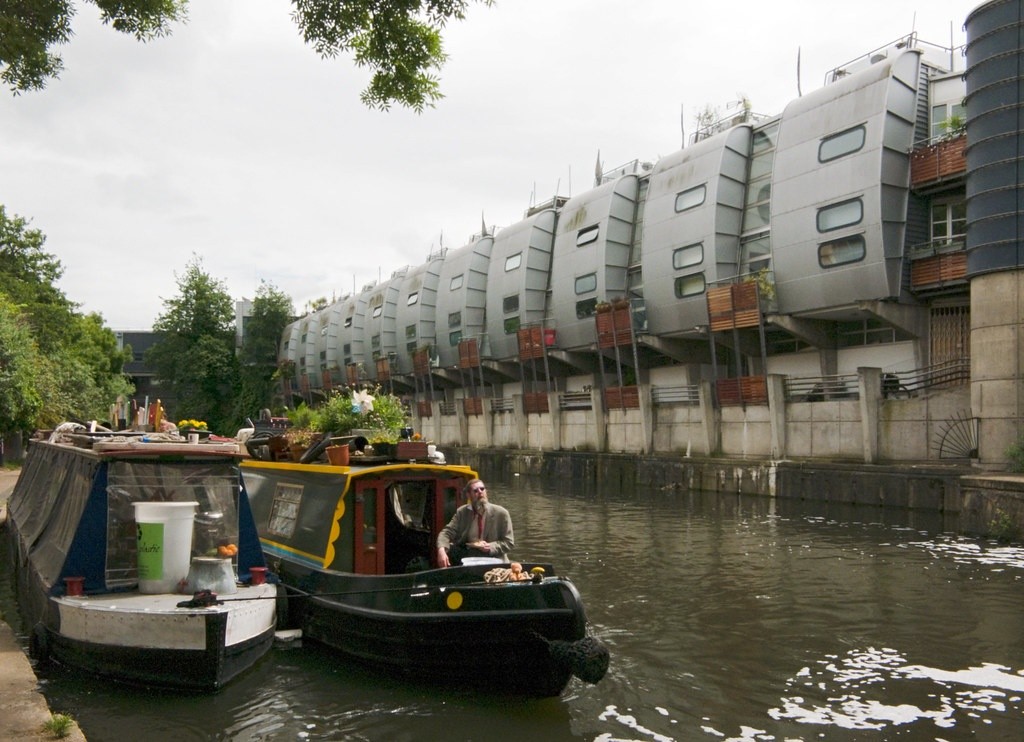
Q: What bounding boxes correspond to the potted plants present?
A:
[367,429,390,455]
[611,297,630,310]
[745,270,773,314]
[269,434,288,460]
[289,401,323,462]
[595,300,610,314]
[326,443,351,467]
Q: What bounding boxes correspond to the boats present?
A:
[4,404,278,691]
[226,399,600,695]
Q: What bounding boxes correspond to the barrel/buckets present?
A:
[131,501,200,596]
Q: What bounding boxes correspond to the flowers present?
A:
[177,419,208,431]
[327,383,423,440]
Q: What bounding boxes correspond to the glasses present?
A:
[471,486,484,494]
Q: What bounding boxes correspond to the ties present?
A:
[475,511,484,540]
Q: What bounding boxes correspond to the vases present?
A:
[179,430,212,440]
[391,441,429,461]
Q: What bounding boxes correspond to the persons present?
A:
[432,479,514,567]
